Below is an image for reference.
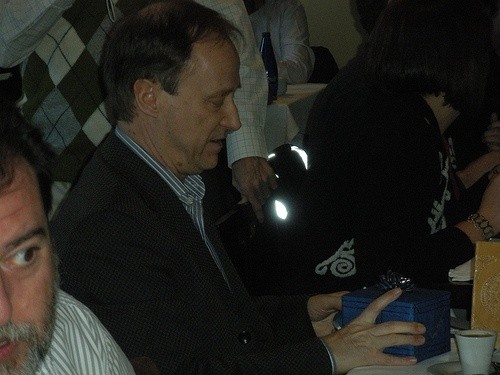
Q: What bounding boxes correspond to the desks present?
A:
[265,83,328,152]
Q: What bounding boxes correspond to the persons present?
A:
[0,103,138,375]
[0,0,499,296]
[46,0,426,375]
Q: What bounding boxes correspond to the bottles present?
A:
[259,31,278,100]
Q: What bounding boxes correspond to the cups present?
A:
[278,79,287,96]
[455,330,497,375]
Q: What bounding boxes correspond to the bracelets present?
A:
[467,212,495,241]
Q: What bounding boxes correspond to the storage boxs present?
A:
[341,274,452,363]
